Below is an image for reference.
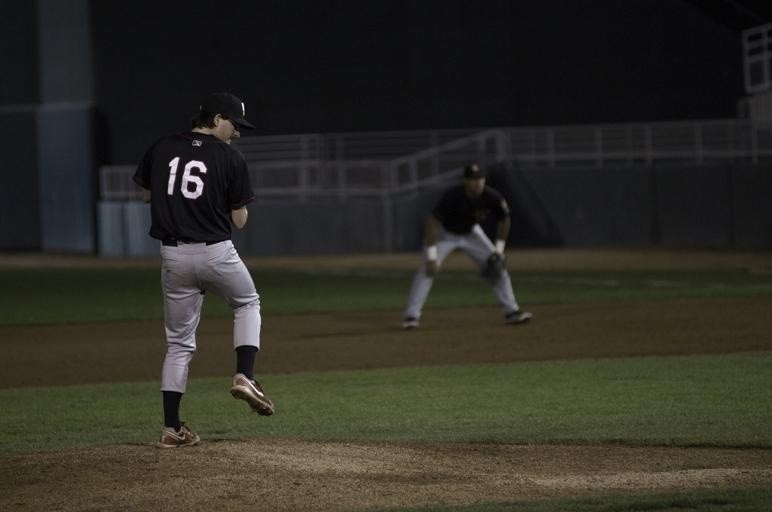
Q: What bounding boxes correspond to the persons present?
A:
[399,155,531,330]
[131,94,274,446]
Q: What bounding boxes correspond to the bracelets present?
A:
[424,245,440,262]
[492,238,507,255]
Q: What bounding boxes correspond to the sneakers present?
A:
[157,419,202,449]
[503,307,534,325]
[228,368,276,418]
[400,314,420,329]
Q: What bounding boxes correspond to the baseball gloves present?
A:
[480,251,506,285]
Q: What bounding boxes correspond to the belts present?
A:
[160,236,231,248]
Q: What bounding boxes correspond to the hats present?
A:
[198,89,258,132]
[462,162,489,180]
